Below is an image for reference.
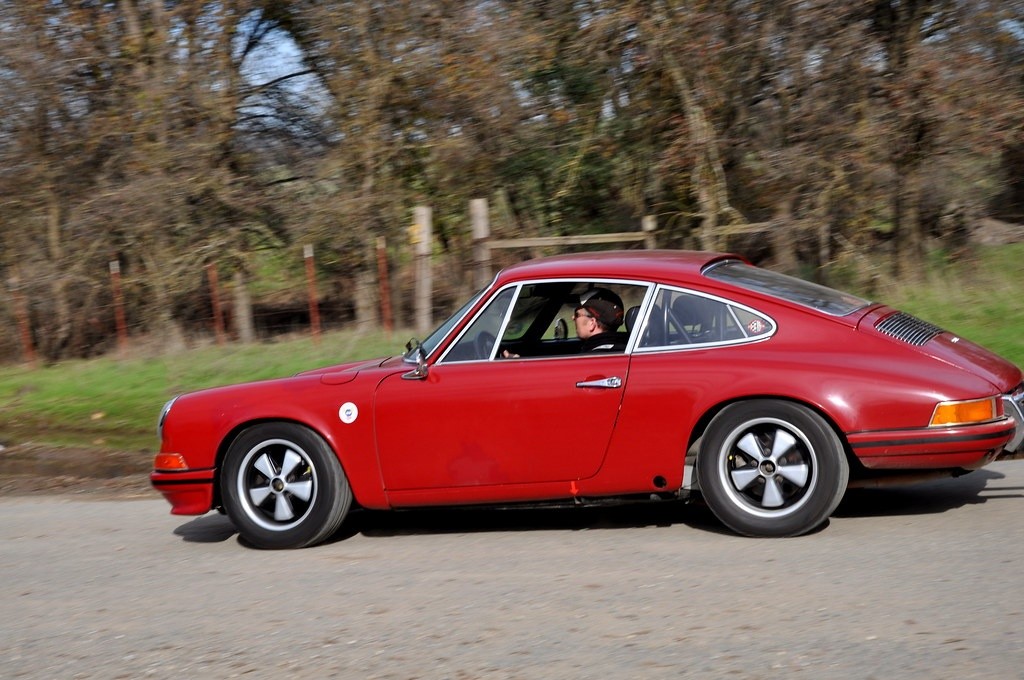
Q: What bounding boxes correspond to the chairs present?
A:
[671,295,717,345]
[624,304,665,347]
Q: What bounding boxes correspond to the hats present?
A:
[558,287,624,325]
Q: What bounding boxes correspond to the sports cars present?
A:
[149,249,1024,551]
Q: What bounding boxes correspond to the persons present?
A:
[503,287,624,360]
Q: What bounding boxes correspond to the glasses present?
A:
[574,312,594,319]
